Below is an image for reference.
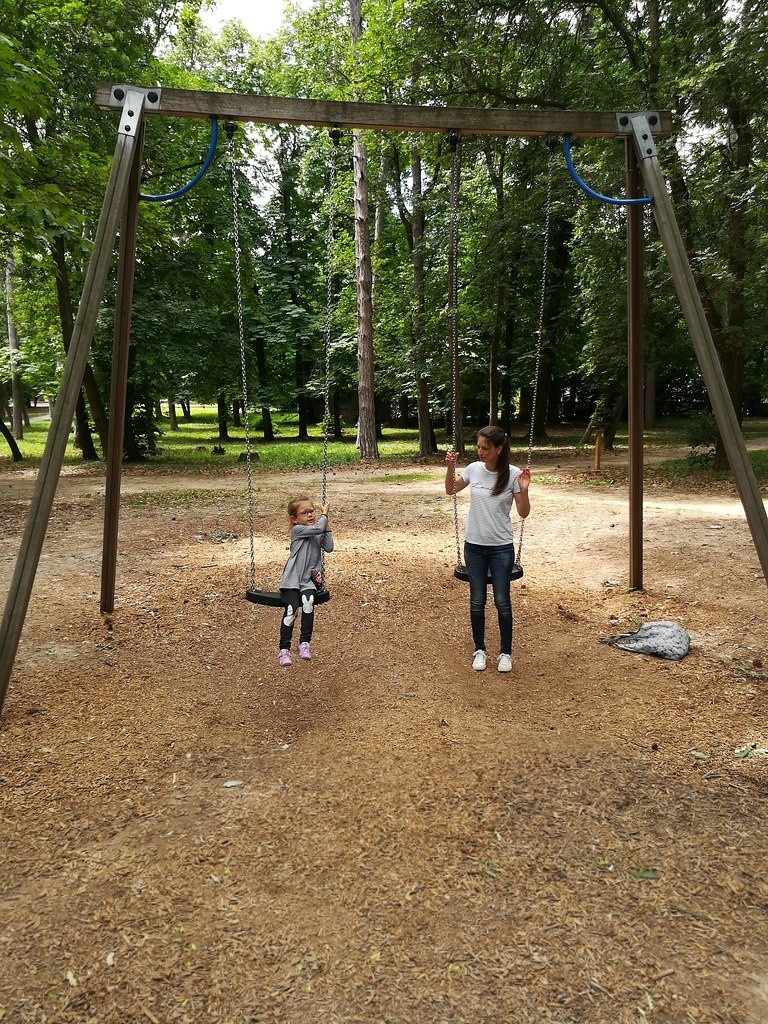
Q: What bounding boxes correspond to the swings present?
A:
[221,122,340,608]
[445,130,558,582]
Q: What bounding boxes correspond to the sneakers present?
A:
[496,653,512,672]
[278,649,293,667]
[472,649,486,670]
[299,641,312,660]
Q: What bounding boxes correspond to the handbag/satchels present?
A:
[609,621,691,661]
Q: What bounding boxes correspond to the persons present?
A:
[445,427,531,673]
[279,498,334,666]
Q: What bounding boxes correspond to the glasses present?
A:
[295,509,315,517]
